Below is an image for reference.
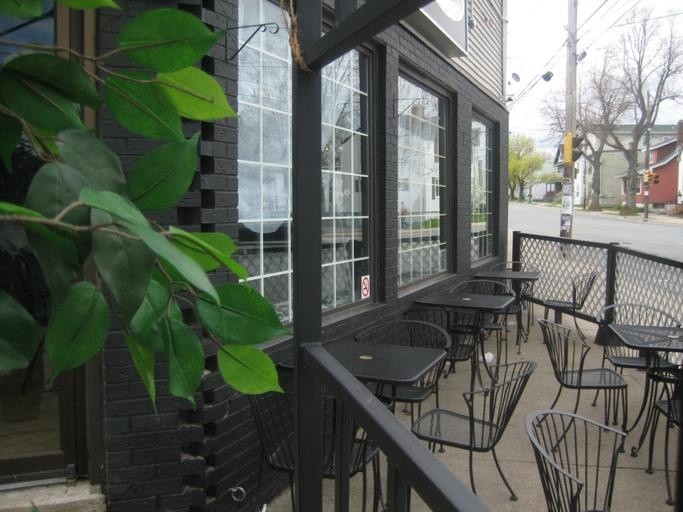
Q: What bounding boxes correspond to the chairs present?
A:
[646,364,681,506]
[536,319,629,455]
[411,359,537,501]
[449,280,516,363]
[488,261,536,325]
[353,320,452,453]
[251,347,385,512]
[478,278,533,338]
[404,306,492,389]
[591,303,682,422]
[542,272,598,345]
[524,410,626,510]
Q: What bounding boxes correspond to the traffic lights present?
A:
[646,170,653,182]
[565,133,584,163]
[652,173,660,184]
